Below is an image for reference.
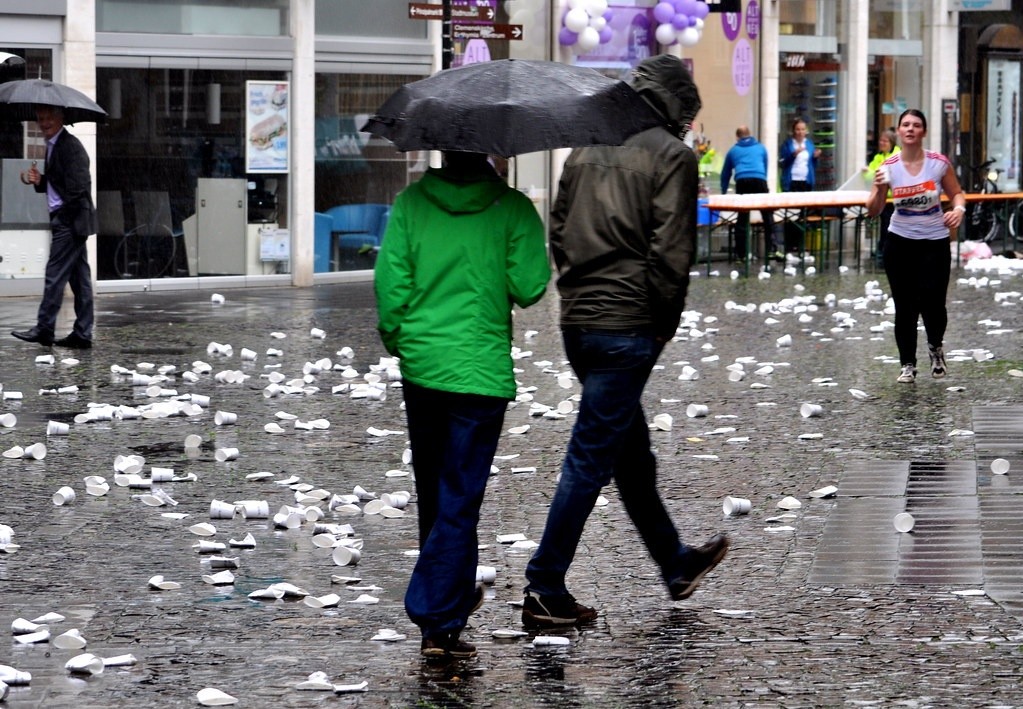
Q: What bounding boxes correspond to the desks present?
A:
[699,191,1023,278]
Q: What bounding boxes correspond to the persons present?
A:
[373,150,553,657]
[720,127,784,264]
[862,131,901,257]
[778,118,822,260]
[865,109,966,383]
[522,53,730,628]
[11,105,102,349]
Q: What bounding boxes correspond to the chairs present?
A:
[313,203,392,272]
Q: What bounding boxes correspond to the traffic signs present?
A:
[451,23,524,41]
[409,2,495,21]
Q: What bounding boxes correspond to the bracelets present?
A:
[952,206,965,212]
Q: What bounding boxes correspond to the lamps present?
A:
[106,78,121,118]
[204,83,221,125]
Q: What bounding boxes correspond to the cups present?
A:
[647,246,1023,449]
[722,495,752,517]
[311,328,326,340]
[211,293,225,305]
[0,343,412,706]
[991,458,1010,475]
[892,512,916,534]
[763,485,837,533]
[878,166,890,184]
[475,331,608,647]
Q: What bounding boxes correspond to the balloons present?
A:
[558,0,613,49]
[651,0,710,49]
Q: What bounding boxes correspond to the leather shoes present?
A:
[11,326,55,347]
[55,332,93,347]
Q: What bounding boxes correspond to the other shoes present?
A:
[767,252,784,260]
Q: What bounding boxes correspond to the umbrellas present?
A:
[0,65,109,185]
[358,58,673,191]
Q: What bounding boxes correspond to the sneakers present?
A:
[470,586,484,615]
[421,632,477,658]
[666,533,728,601]
[897,363,916,383]
[523,589,597,629]
[929,349,947,379]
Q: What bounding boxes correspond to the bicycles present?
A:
[955,157,1023,244]
